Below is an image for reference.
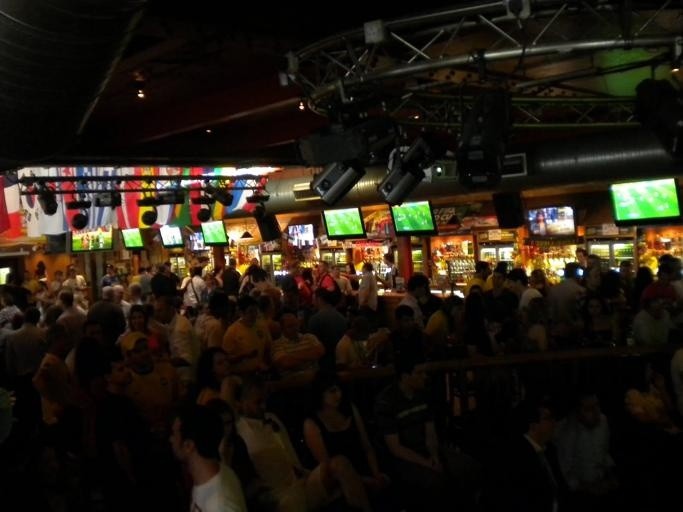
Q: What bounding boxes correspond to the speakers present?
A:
[492,187,525,229]
[224,212,281,243]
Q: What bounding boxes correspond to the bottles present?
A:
[590,246,632,271]
[483,250,514,275]
[625,330,634,354]
[447,253,475,274]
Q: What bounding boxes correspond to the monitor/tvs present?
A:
[287,224,315,250]
[66,223,113,254]
[389,200,438,236]
[121,228,144,250]
[159,224,184,247]
[322,206,367,240]
[525,203,577,241]
[609,177,681,227]
[200,220,229,246]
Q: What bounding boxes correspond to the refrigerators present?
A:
[318,248,351,273]
[168,255,186,275]
[258,251,284,274]
[585,236,638,279]
[389,244,424,275]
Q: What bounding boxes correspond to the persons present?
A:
[325,210,359,237]
[530,211,551,235]
[81,232,106,251]
[1,246,683,511]
[391,205,435,230]
[611,184,678,221]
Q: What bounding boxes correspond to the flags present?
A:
[1,165,279,241]
